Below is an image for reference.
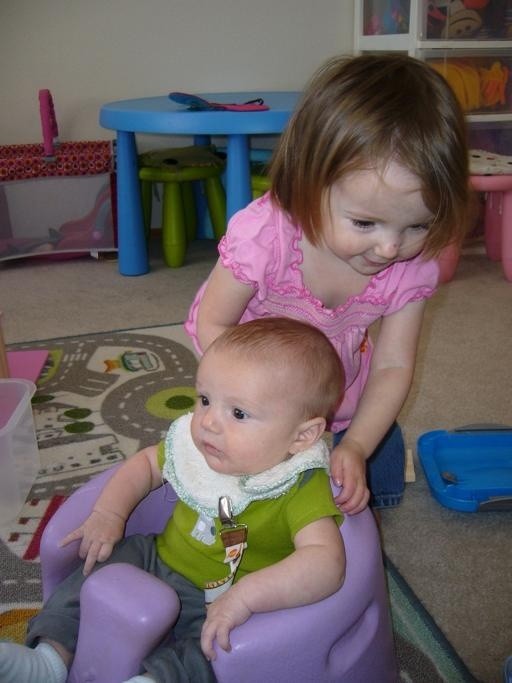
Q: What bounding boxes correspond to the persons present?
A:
[187,51,483,528]
[0,317,349,683]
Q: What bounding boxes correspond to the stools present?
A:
[433,174,512,283]
[135,146,228,267]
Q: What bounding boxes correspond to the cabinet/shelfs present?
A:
[352,0,512,247]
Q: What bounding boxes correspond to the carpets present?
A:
[0,318,495,682]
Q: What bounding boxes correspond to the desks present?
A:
[98,91,309,279]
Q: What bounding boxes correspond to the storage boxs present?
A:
[0,376,41,527]
